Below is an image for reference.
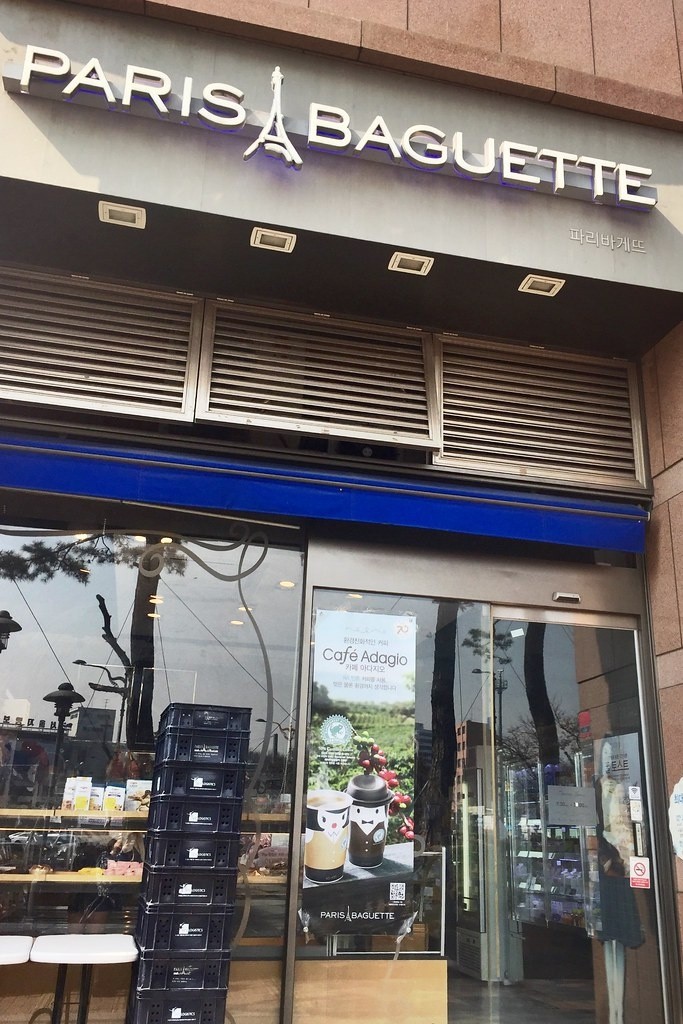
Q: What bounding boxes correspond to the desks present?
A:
[0,933,138,1024]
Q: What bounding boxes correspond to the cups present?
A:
[306,789,354,885]
[342,774,393,870]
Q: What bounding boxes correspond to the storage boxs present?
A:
[125,704,253,1024]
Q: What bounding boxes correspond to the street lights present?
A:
[39,683,84,874]
[74,659,127,746]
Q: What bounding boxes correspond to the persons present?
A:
[67,846,112,934]
[593,737,646,1024]
[108,832,145,863]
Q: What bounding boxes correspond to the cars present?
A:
[8,833,20,839]
[11,832,38,843]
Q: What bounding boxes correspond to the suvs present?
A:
[38,833,79,860]
